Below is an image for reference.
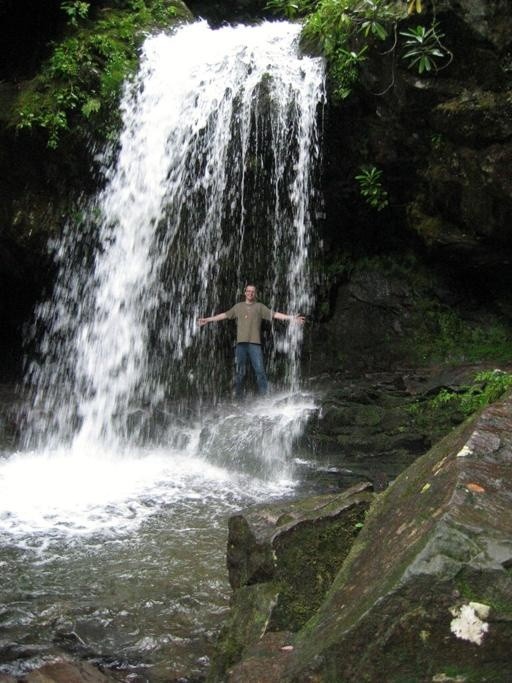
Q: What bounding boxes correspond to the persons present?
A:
[195,285,307,404]
